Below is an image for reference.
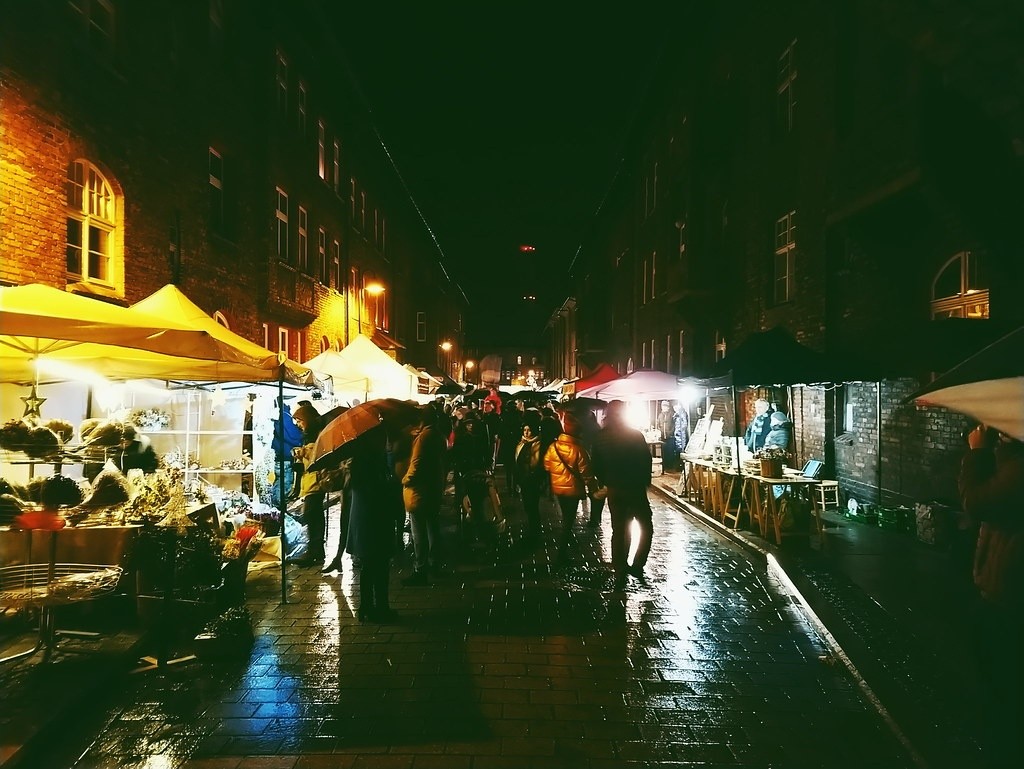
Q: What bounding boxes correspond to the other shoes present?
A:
[290,551,326,567]
[359,608,398,621]
[628,563,649,586]
[400,574,429,587]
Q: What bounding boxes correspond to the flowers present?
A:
[124,406,173,431]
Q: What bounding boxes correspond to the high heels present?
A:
[321,558,343,573]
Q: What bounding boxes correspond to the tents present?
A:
[0,283,1024,647]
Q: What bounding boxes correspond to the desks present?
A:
[648,441,664,475]
[681,453,823,545]
[0,525,144,588]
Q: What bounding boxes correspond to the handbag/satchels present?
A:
[575,477,587,500]
[319,470,345,492]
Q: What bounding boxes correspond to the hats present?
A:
[461,412,481,424]
[564,413,583,435]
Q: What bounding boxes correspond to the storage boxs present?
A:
[844,504,916,536]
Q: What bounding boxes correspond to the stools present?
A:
[815,480,839,511]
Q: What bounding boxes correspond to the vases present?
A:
[132,421,161,432]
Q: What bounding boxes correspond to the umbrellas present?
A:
[306,380,609,475]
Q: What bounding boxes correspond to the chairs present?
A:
[798,460,825,501]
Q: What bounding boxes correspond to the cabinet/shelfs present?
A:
[121,387,262,505]
[0,443,128,698]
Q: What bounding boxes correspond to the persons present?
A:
[959,423,1024,605]
[113,421,159,477]
[271,386,653,622]
[743,398,793,503]
[658,400,689,471]
